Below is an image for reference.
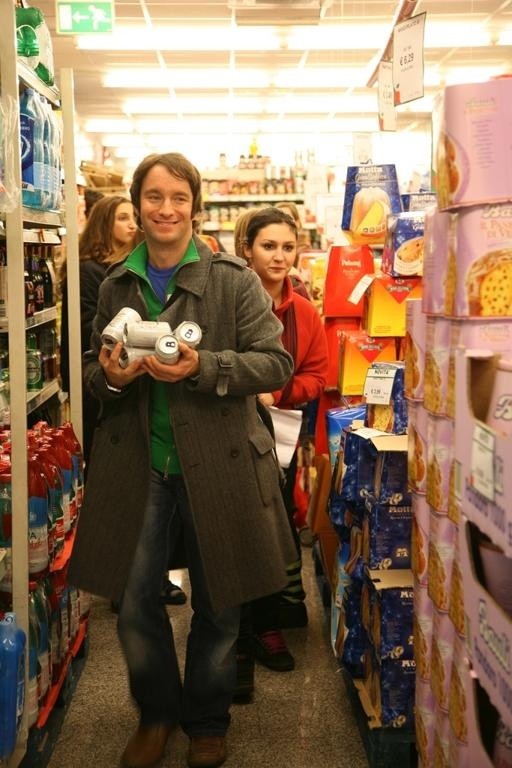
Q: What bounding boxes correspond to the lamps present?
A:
[72,13,512,156]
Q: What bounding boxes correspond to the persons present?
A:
[229,208,330,708]
[192,202,313,304]
[53,150,300,768]
[58,192,189,615]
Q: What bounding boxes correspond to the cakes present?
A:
[393,134,512,768]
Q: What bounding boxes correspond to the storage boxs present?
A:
[292,78,510,768]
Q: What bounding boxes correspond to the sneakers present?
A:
[188,736,227,768]
[255,630,294,671]
[162,577,186,605]
[118,716,177,768]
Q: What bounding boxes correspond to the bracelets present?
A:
[104,378,123,394]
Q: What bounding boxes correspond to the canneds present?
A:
[100,306,203,368]
[0,328,58,406]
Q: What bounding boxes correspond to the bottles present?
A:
[16,7,54,86]
[0,421,84,582]
[0,244,56,319]
[19,88,62,211]
[0,564,91,762]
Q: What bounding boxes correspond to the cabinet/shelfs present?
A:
[76,157,332,272]
[0,1,85,766]
